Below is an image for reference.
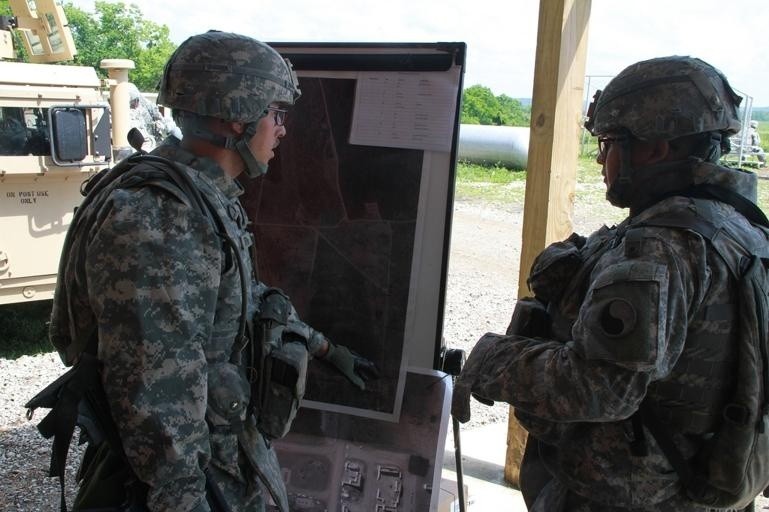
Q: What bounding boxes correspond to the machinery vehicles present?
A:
[0,0,181,308]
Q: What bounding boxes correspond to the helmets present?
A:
[584,54,743,142]
[157,29,302,123]
[128,83,140,108]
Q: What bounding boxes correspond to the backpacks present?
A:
[618,203,769,512]
[47,155,231,369]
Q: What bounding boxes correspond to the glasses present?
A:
[265,104,289,126]
[597,135,639,156]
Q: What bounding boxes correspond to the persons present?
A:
[449,55,769,512]
[736,120,767,168]
[48,28,382,511]
[106,83,176,163]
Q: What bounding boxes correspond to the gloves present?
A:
[324,340,380,391]
